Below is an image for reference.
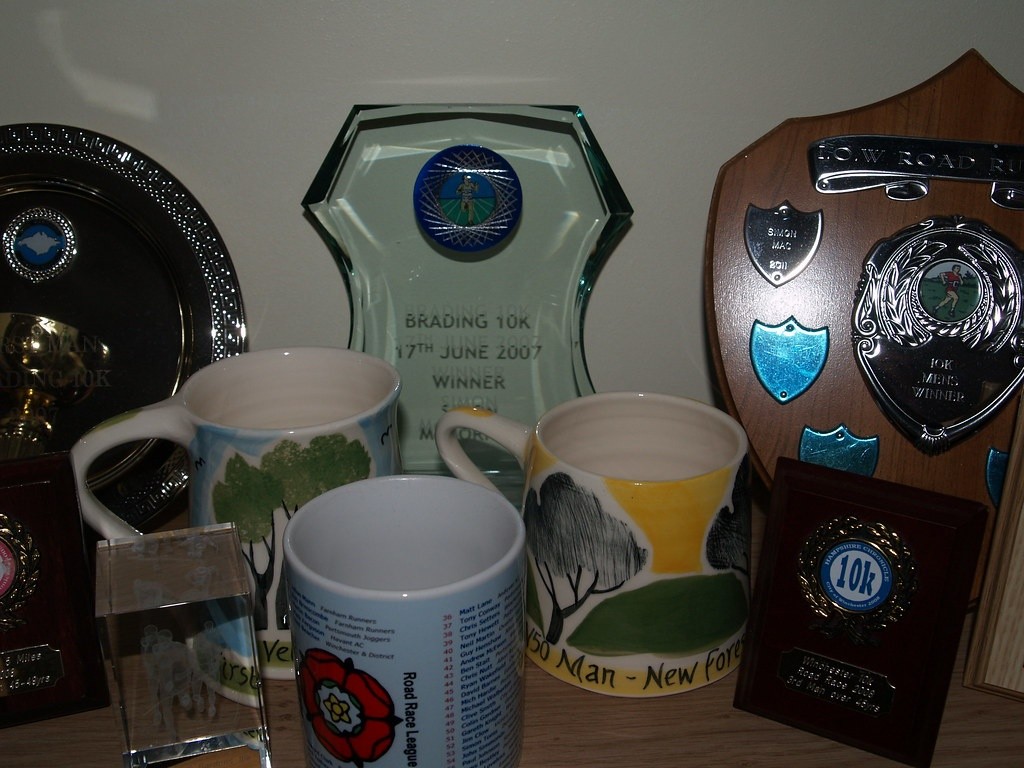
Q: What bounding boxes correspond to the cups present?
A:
[70,348,402,680]
[435,393,750,698]
[282,476,527,768]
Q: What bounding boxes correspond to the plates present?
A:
[0,123,247,559]
